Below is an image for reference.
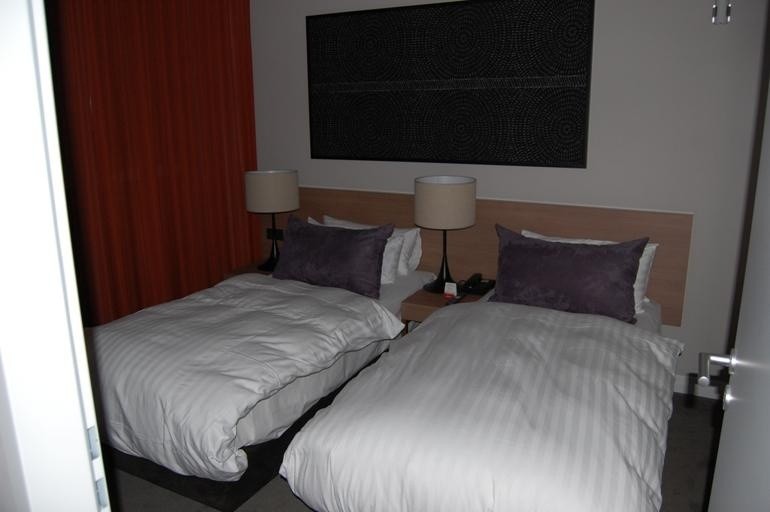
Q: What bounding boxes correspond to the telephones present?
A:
[462,273,496,295]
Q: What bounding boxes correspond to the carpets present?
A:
[103,350,340,512]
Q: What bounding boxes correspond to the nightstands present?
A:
[401,286,491,334]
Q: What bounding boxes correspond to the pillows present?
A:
[487,223,658,326]
[271,215,417,299]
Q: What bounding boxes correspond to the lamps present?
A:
[413,175,477,293]
[243,170,300,270]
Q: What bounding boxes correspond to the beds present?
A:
[277,287,686,512]
[84,215,423,482]
